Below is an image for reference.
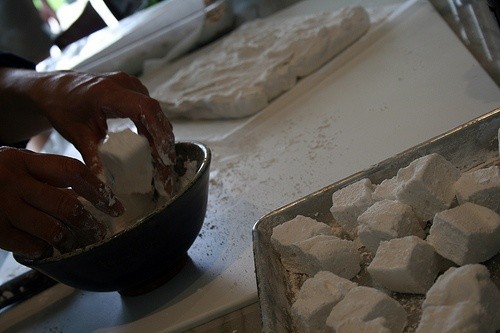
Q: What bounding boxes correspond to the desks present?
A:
[0,0,500,333]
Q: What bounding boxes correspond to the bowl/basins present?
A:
[12,139,212,299]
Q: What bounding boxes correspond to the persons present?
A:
[0,0,176,260]
